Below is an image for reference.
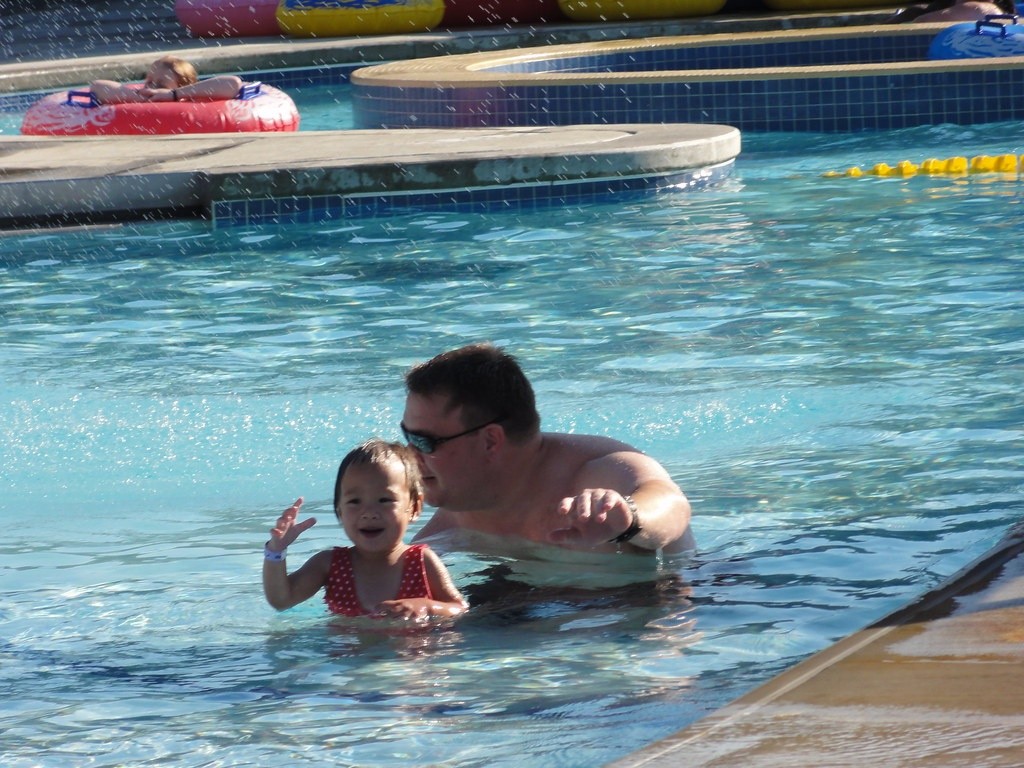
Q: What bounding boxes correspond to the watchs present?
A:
[607,495,643,547]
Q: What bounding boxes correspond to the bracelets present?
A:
[170,87,177,100]
[264,542,288,560]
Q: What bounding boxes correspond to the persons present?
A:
[261,442,468,624]
[87,57,242,103]
[399,347,700,554]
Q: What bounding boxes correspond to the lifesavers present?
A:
[21,81,301,135]
[175,0,898,42]
[928,13,1024,61]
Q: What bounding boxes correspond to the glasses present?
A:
[400,420,494,455]
